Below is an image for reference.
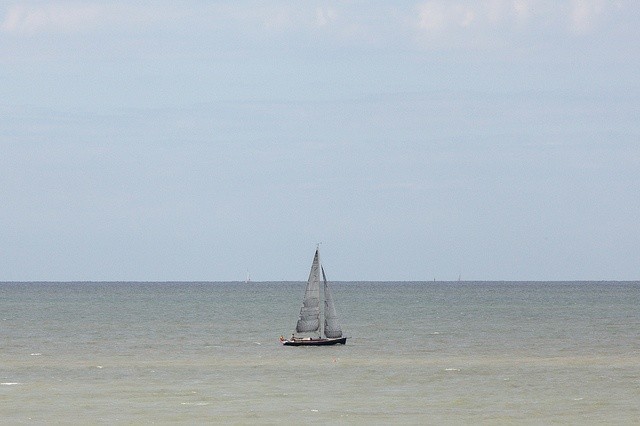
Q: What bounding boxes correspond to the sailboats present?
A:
[279,249,346,346]
